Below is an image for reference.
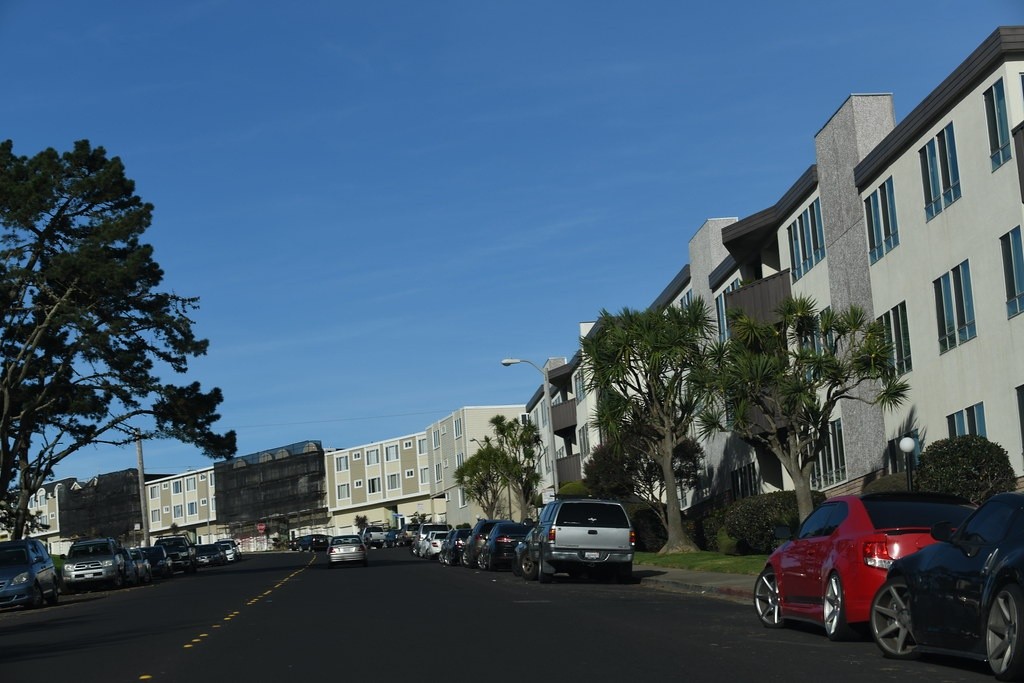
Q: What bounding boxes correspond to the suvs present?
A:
[519,494,635,584]
[297,534,328,553]
[153,532,194,571]
[60,537,127,595]
[219,540,240,559]
[461,519,514,569]
[397,524,420,546]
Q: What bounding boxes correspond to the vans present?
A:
[412,523,449,556]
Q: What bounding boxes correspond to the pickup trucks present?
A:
[359,526,389,549]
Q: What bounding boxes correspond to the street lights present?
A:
[501,358,560,501]
[899,436,916,491]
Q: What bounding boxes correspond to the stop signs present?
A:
[258,523,265,531]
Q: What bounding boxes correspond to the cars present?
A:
[753,492,978,642]
[438,528,458,564]
[326,535,368,569]
[383,529,401,548]
[120,548,139,587]
[139,545,172,580]
[219,543,237,563]
[477,522,535,572]
[421,531,443,559]
[289,536,311,551]
[868,492,1024,682]
[0,538,60,609]
[129,549,152,584]
[194,544,226,568]
[511,527,535,577]
[429,532,450,560]
[444,527,474,566]
